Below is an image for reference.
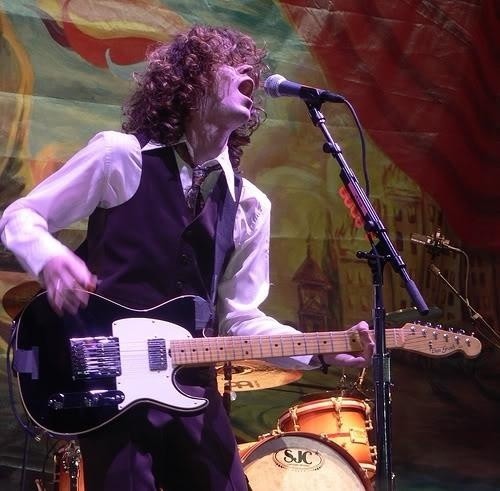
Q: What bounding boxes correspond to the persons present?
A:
[0,18,375,491]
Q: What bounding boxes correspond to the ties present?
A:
[174,142,222,220]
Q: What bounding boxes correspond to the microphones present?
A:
[430,263,441,276]
[411,228,464,256]
[264,74,345,103]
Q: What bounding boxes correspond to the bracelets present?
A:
[316,354,332,374]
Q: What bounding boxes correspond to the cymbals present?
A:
[300,391,365,401]
[214,360,304,394]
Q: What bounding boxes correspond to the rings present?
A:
[55,277,62,291]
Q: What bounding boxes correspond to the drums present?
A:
[236,429,373,491]
[58,446,85,491]
[277,398,377,481]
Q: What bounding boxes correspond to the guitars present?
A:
[12,287,482,438]
